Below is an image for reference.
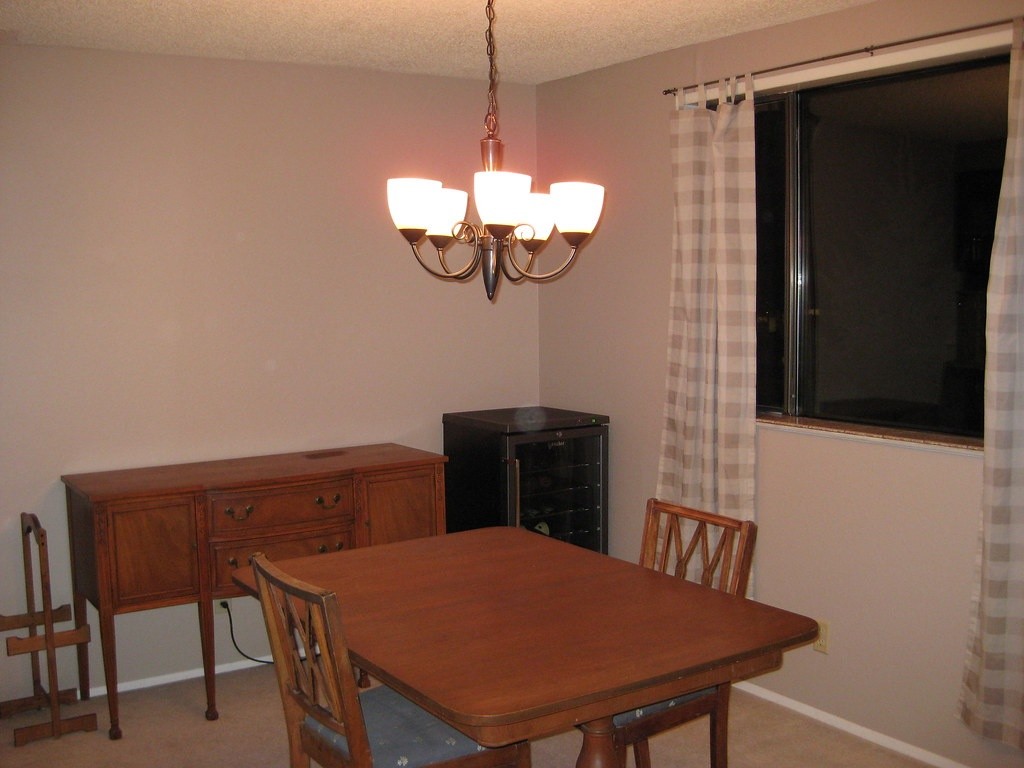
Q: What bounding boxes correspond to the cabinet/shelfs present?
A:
[442,406,611,559]
[60,441,449,744]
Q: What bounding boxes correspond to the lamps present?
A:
[385,0,608,305]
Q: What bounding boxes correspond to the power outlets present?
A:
[215,598,232,615]
[813,620,831,655]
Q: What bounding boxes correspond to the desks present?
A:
[228,526,822,768]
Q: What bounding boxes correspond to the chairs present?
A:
[611,496,761,768]
[253,554,535,768]
